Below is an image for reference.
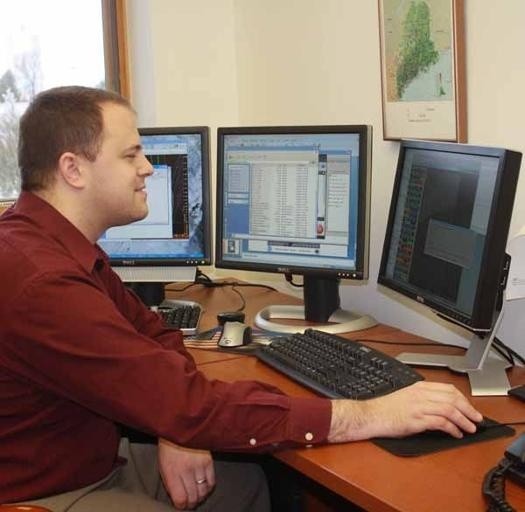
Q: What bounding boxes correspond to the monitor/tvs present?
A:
[95,126,214,312]
[376,138,524,396]
[214,124,380,335]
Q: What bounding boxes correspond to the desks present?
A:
[164,277,525,512]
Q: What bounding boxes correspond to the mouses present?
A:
[218,321,252,347]
[434,419,485,439]
[218,311,247,327]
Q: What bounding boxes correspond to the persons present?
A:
[0,87,483,512]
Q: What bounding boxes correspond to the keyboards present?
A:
[145,304,202,335]
[254,329,425,401]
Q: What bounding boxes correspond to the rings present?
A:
[195,478,207,484]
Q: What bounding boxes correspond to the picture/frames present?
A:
[375,0,468,143]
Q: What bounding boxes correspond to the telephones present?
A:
[497,433,525,484]
